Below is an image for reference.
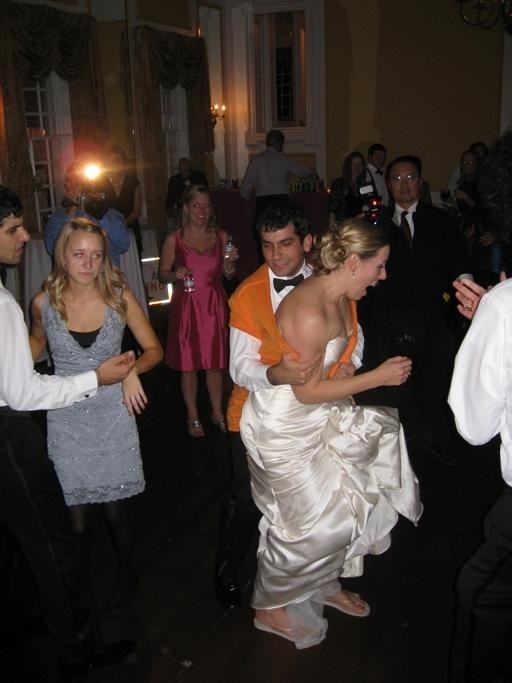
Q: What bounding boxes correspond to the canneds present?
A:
[291,168,324,193]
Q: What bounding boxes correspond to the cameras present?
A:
[358,182,384,229]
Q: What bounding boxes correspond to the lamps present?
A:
[211,104,227,127]
[455,0,512,37]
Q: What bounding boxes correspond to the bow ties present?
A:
[273,274,304,294]
[376,168,384,175]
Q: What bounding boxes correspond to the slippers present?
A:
[187,418,206,439]
[252,617,319,643]
[211,417,228,433]
[311,590,371,618]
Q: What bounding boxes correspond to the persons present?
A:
[2,130,511,651]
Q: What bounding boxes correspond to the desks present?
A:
[207,188,330,271]
[23,228,148,332]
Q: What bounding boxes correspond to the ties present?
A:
[401,211,412,248]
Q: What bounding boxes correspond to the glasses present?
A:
[389,174,420,184]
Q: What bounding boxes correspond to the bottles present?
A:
[183,271,196,292]
[287,179,325,193]
[223,244,233,259]
[220,174,239,191]
